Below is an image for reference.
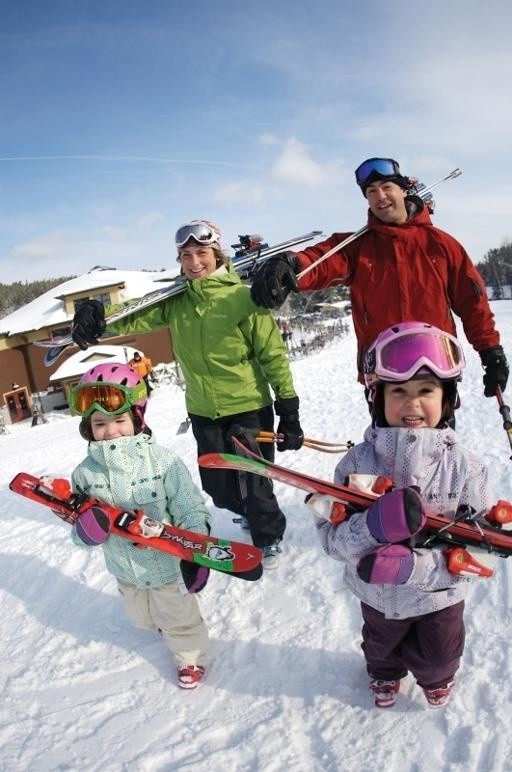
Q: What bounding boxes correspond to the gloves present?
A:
[367,485,427,543]
[355,544,416,585]
[180,557,210,594]
[70,300,108,349]
[250,251,302,309]
[274,396,304,452]
[478,344,510,398]
[74,507,112,549]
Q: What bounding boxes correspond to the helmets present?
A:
[364,322,462,386]
[79,361,148,407]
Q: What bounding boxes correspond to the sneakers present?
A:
[424,676,457,709]
[261,541,282,570]
[370,678,402,707]
[177,662,205,689]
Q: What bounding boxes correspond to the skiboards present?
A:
[272,168,462,297]
[32,228,323,376]
[196,451,511,558]
[8,472,263,580]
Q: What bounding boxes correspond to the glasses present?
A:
[67,382,142,415]
[356,158,405,186]
[174,222,218,248]
[374,327,466,383]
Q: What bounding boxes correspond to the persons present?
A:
[71,219,304,569]
[127,352,156,398]
[250,158,510,431]
[314,323,499,709]
[70,363,212,688]
